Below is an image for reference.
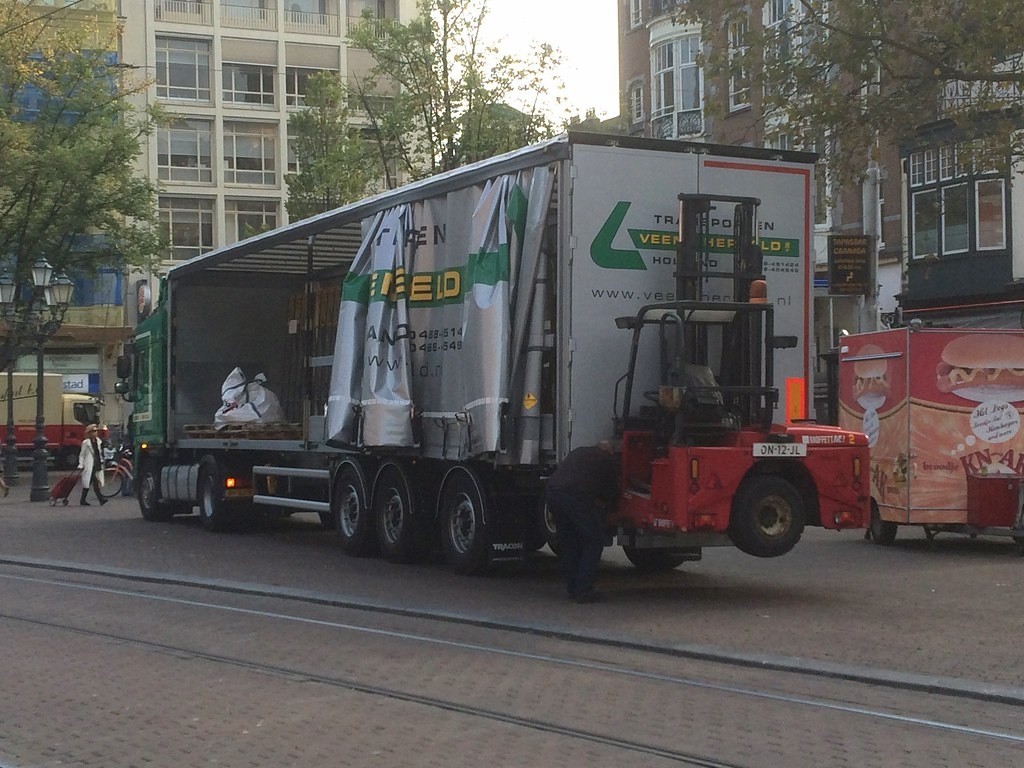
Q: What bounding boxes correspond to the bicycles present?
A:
[97,445,135,497]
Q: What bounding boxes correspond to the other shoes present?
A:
[571,591,598,603]
[101,499,108,505]
[80,501,90,505]
[4,487,9,497]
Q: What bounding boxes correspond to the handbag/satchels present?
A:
[214,366,286,432]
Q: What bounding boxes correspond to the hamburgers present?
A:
[936,334,1024,404]
[852,345,890,410]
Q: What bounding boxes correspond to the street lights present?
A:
[0,250,74,503]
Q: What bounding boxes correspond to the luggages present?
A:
[48,468,82,507]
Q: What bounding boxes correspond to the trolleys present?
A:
[49,468,84,506]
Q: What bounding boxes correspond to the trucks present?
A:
[0,371,111,470]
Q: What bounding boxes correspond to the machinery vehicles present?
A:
[600,187,872,558]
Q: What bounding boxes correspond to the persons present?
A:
[77,423,108,505]
[0,470,9,497]
[543,440,621,603]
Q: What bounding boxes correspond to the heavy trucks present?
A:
[114,129,821,571]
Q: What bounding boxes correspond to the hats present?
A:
[85,424,97,433]
